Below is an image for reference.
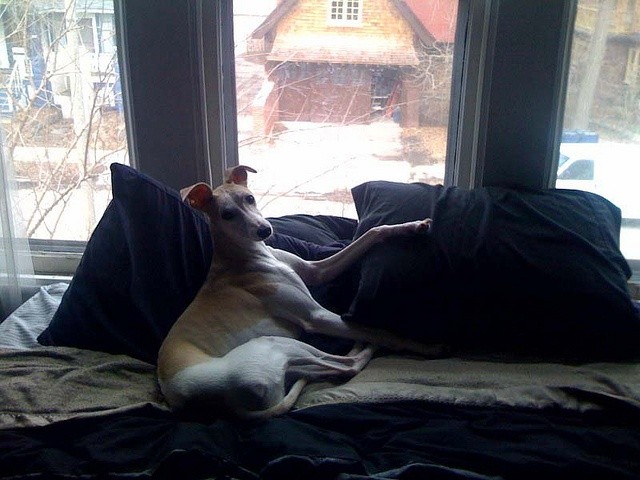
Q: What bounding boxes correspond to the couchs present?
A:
[0,255,640,479]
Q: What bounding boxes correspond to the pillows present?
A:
[352,180,640,362]
[266,215,358,245]
[37,163,351,366]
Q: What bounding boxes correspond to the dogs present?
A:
[155,163,436,421]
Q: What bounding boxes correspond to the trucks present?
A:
[554,143,639,220]
